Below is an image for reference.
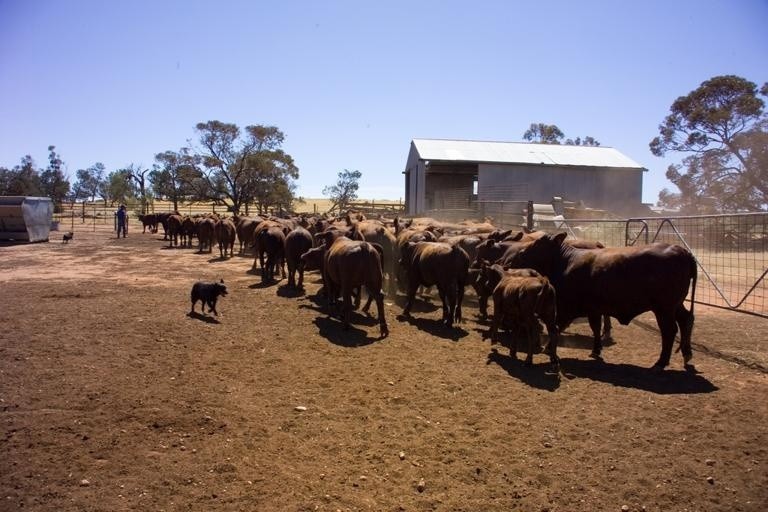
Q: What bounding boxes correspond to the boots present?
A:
[118,234,128,238]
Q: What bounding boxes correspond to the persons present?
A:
[117,204,127,239]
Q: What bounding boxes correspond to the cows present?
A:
[482,265,560,367]
[137,212,237,258]
[232,208,605,293]
[517,229,699,368]
[312,229,388,341]
[396,238,471,327]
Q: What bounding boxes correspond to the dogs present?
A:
[189,280,230,318]
[62,230,74,243]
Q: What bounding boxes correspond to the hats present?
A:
[121,205,127,208]
